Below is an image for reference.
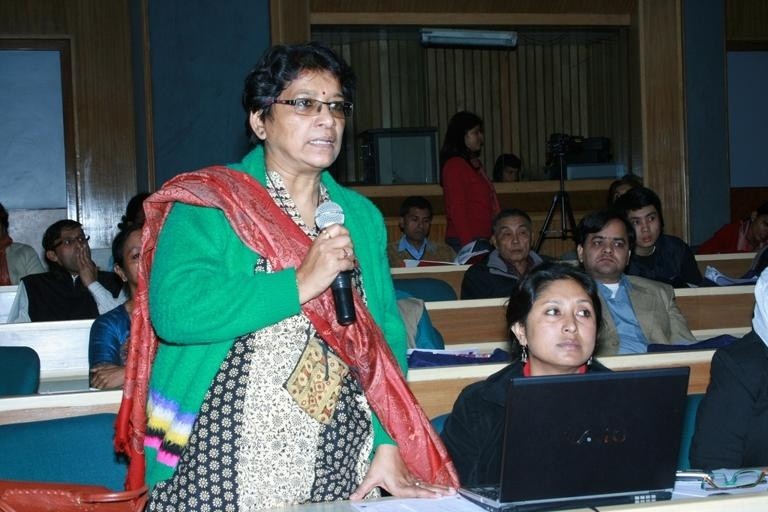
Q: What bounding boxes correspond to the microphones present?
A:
[316,201,360,325]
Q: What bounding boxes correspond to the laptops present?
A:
[458,366,691,511]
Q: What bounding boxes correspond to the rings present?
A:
[415,482,420,486]
[322,228,331,239]
[342,248,349,259]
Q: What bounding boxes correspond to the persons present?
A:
[89,223,144,390]
[118,192,154,231]
[386,178,768,302]
[688,265,768,474]
[439,261,616,489]
[439,111,502,255]
[114,40,462,510]
[394,289,445,351]
[0,203,47,287]
[6,219,132,324]
[575,209,698,358]
[493,154,522,182]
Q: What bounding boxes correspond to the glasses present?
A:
[51,234,90,251]
[273,98,354,119]
[700,469,767,491]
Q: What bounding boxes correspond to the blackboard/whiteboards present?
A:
[0,47,69,209]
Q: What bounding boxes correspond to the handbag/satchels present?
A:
[0,480,149,512]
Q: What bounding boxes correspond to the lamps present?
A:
[419,28,518,47]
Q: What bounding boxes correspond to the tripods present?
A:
[534,160,579,254]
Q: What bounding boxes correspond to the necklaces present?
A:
[265,168,321,235]
